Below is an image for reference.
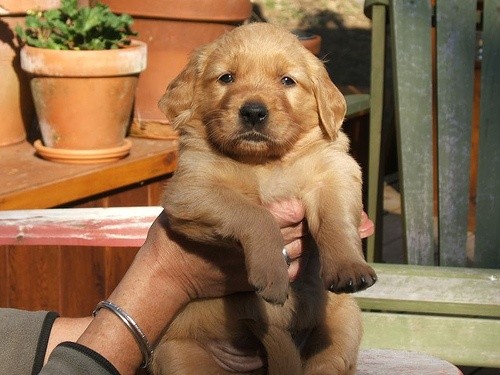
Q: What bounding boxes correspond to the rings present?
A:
[280,246,293,269]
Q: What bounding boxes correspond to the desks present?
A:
[0,138,171,208]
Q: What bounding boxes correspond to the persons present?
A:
[0,195,318,375]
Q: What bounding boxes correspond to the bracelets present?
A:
[91,300,154,370]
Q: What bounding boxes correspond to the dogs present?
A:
[145,20,379,375]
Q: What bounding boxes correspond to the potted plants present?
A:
[12,1,148,161]
